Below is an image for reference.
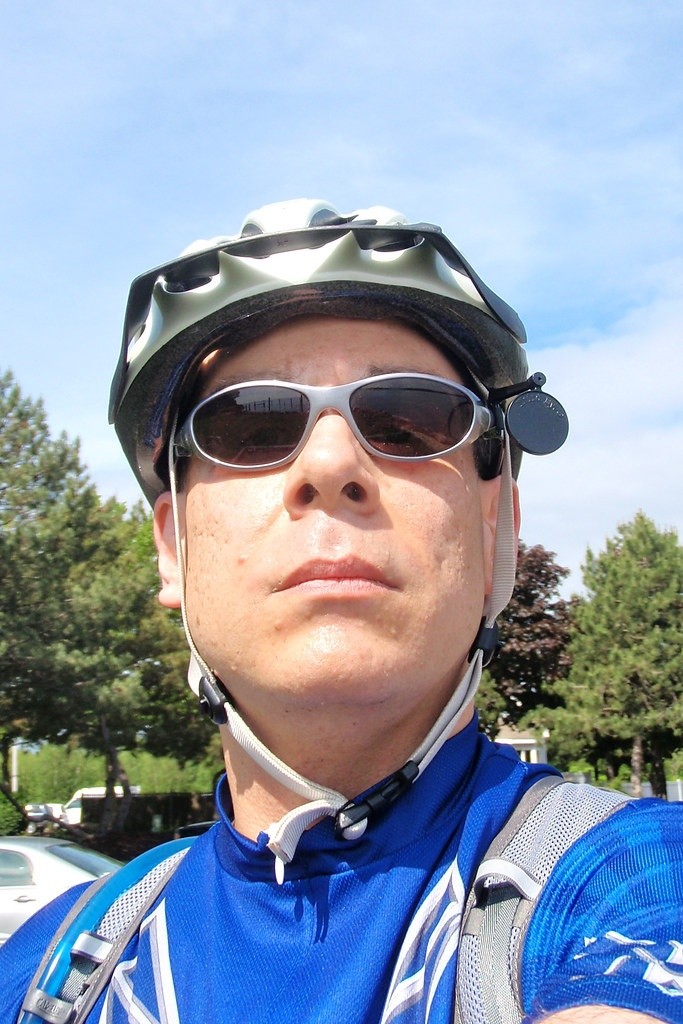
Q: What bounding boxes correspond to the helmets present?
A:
[109,200,530,508]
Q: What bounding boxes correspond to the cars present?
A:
[0,834,128,947]
[25,803,66,836]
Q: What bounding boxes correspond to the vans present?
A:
[64,786,142,825]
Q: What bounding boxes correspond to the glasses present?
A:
[172,373,494,471]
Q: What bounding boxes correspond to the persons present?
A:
[0,200,682,1024]
[174,792,219,838]
